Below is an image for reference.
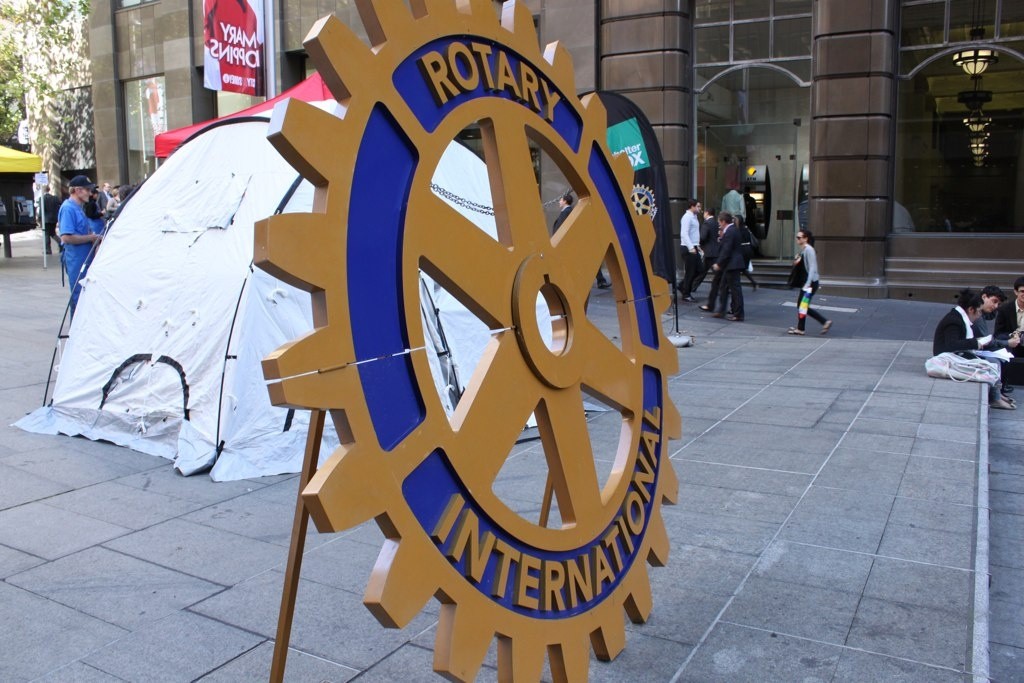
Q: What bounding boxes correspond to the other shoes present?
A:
[1001,385,1014,392]
[46,251,52,254]
[820,320,832,335]
[699,305,714,312]
[677,285,685,294]
[1000,395,1017,404]
[990,399,1017,410]
[727,310,734,314]
[682,296,697,302]
[753,283,759,291]
[728,316,744,320]
[710,313,725,317]
[787,328,805,335]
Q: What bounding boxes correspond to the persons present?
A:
[788,231,833,334]
[203,0,259,96]
[933,291,1016,409]
[553,195,573,235]
[39,186,63,254]
[678,182,758,320]
[84,182,136,235]
[58,175,103,324]
[972,286,1020,391]
[596,268,612,288]
[993,276,1024,360]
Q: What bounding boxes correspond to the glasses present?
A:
[796,236,804,240]
[1018,290,1024,293]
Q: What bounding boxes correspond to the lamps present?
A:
[952,0,999,81]
[957,79,993,169]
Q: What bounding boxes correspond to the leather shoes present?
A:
[598,282,612,289]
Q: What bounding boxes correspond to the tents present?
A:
[10,99,607,481]
[154,71,336,170]
[0,146,47,268]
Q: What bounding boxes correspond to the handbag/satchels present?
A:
[924,354,1000,386]
[745,226,763,255]
[788,261,809,288]
[745,260,753,272]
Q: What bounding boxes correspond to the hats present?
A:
[68,174,95,187]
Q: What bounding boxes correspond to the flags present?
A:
[577,90,676,283]
